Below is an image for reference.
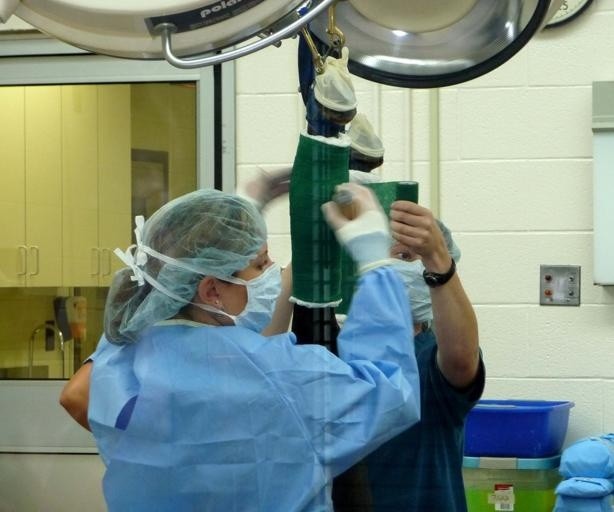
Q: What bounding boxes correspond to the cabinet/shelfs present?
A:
[1,84,135,289]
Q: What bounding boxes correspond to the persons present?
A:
[83,162,422,512]
[58,353,95,431]
[259,198,487,511]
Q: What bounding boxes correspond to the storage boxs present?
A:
[463,398,575,457]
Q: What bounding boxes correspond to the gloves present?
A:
[313,47,358,111]
[238,165,291,211]
[321,182,392,273]
[348,113,385,158]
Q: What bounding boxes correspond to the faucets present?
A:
[29,322,66,380]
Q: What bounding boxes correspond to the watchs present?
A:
[422,258,456,287]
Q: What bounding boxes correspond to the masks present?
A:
[234,262,283,332]
[394,259,436,326]
